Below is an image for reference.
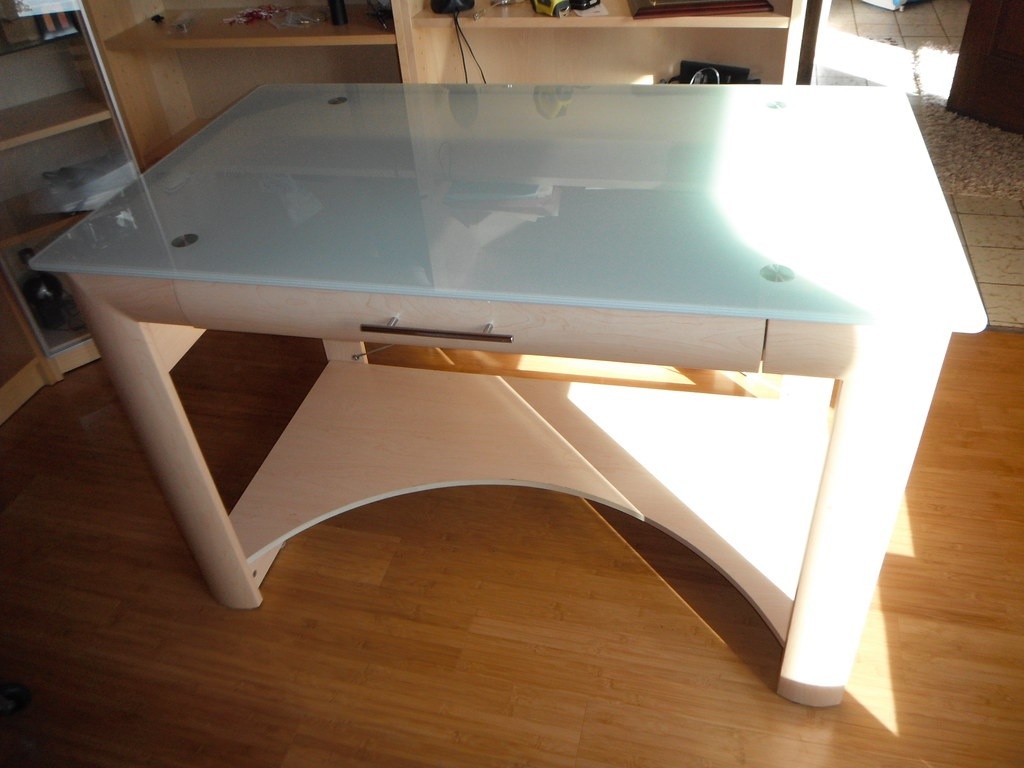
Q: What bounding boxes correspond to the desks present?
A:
[28,81,988,709]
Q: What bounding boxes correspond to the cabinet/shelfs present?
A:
[401,0,813,303]
[0,11,140,381]
[81,0,441,288]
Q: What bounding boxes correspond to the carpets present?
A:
[814,35,1024,201]
